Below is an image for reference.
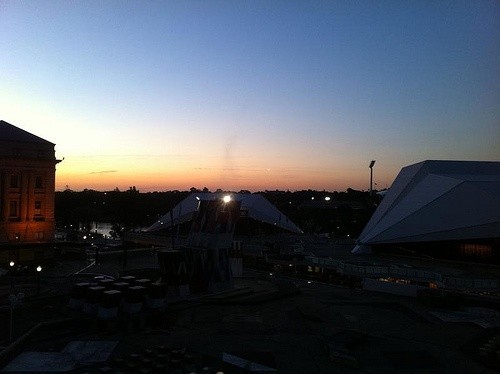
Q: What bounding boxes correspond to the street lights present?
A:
[369,160,375,190]
